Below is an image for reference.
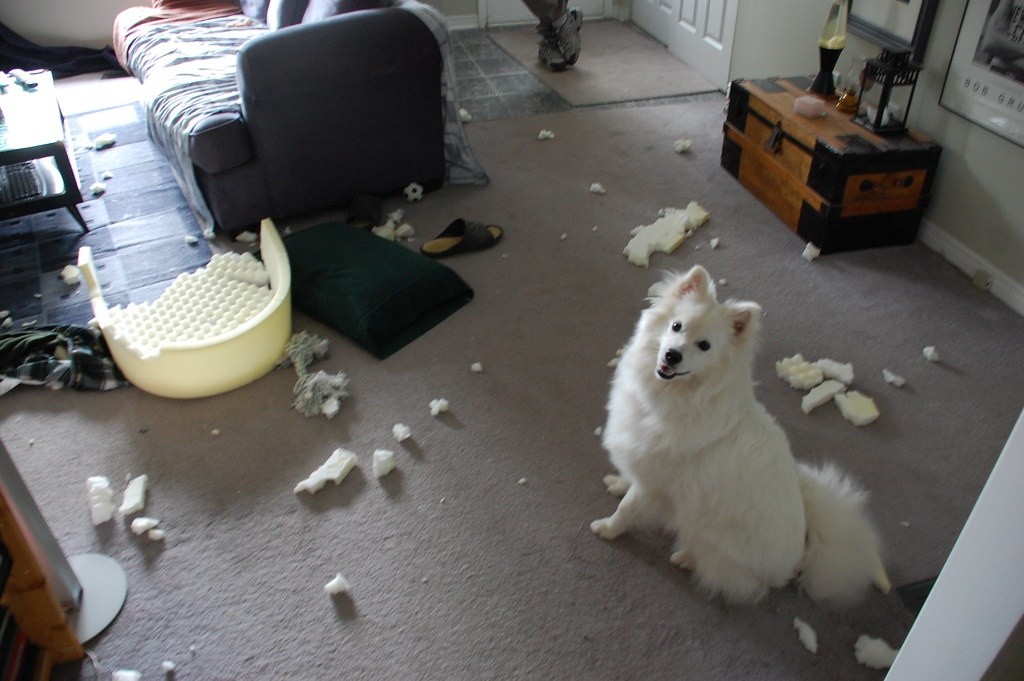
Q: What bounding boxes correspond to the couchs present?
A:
[112,0,447,238]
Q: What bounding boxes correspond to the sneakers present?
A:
[538,38,567,72]
[557,8,584,65]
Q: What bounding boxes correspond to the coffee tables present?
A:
[0,69,93,235]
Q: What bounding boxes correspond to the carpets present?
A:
[486,19,720,108]
[0,101,215,329]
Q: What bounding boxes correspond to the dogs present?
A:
[590,264,876,619]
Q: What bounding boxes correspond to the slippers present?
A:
[420,217,504,259]
[346,197,382,235]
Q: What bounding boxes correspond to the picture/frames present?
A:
[845,0,940,66]
[937,0,1024,149]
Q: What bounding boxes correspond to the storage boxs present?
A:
[720,76,945,255]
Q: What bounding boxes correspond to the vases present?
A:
[806,0,849,100]
